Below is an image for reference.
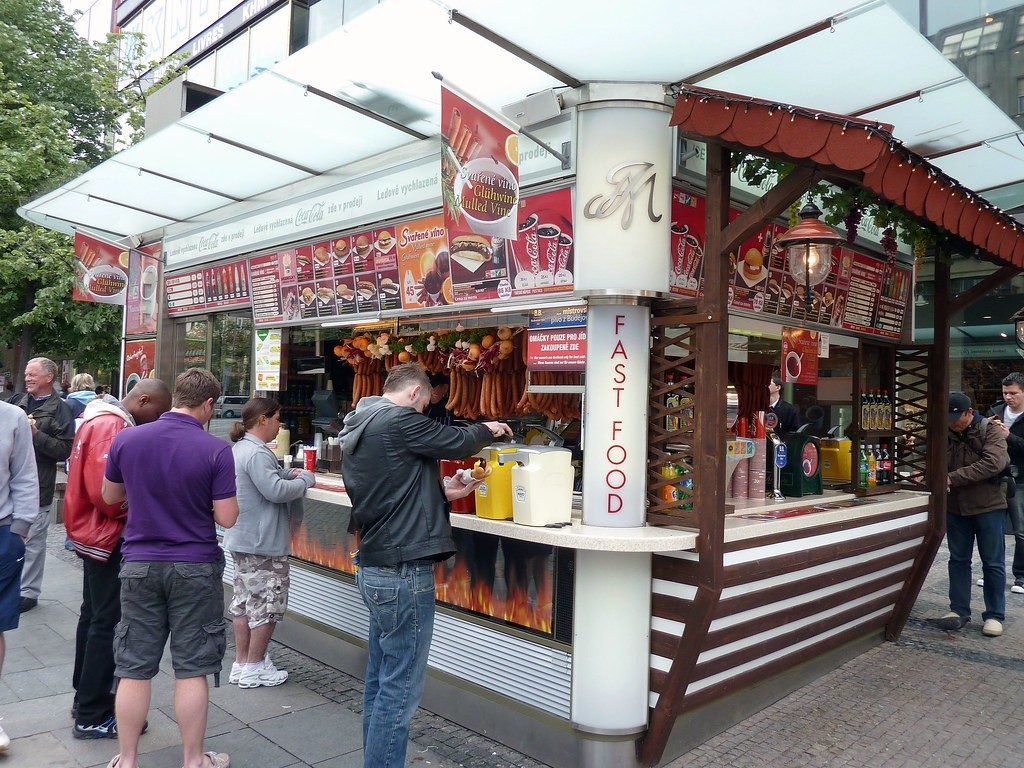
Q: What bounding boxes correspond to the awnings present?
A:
[669,83,1024,270]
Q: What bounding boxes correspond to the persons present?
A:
[3,356,75,613]
[769,376,798,432]
[223,398,316,688]
[0,374,13,401]
[101,366,240,768]
[63,372,98,551]
[338,362,512,768]
[977,372,1024,594]
[0,399,39,749]
[62,378,171,739]
[941,392,1010,636]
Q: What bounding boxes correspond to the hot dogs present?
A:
[450,235,492,262]
[471,460,492,480]
[357,280,376,295]
[318,288,334,298]
[782,283,793,296]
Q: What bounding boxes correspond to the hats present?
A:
[948,391,971,420]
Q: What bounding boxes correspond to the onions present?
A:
[334,326,512,361]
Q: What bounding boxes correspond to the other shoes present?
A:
[19,597,37,612]
[204,751,230,768]
[0,717,10,750]
[107,754,140,768]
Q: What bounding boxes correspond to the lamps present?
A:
[1009,309,1024,358]
[772,192,848,314]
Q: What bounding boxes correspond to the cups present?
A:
[555,233,573,273]
[454,158,520,242]
[139,266,158,315]
[83,265,128,305]
[313,432,324,460]
[512,213,539,277]
[668,220,703,283]
[302,449,317,473]
[535,223,561,278]
[735,409,768,500]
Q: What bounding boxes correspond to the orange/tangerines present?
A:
[442,277,453,303]
[504,134,518,166]
[118,252,128,268]
[149,369,154,378]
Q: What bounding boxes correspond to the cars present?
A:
[214,396,250,418]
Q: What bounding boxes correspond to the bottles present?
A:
[859,389,893,487]
[206,263,247,297]
[276,364,316,435]
[761,229,772,265]
[882,266,909,301]
[491,233,505,265]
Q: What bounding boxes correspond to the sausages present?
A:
[351,330,582,421]
[338,290,354,295]
[768,283,779,292]
[381,283,398,291]
[728,362,774,416]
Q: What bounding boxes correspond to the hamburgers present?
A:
[333,230,392,257]
[825,292,832,305]
[744,248,763,279]
[302,287,313,303]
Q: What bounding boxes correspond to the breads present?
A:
[769,279,777,284]
[337,284,347,290]
[797,287,804,295]
[381,278,392,284]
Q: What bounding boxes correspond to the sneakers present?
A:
[238,653,288,688]
[229,662,246,684]
[942,612,966,628]
[983,618,1002,635]
[977,578,984,586]
[1010,582,1024,593]
[72,715,148,740]
